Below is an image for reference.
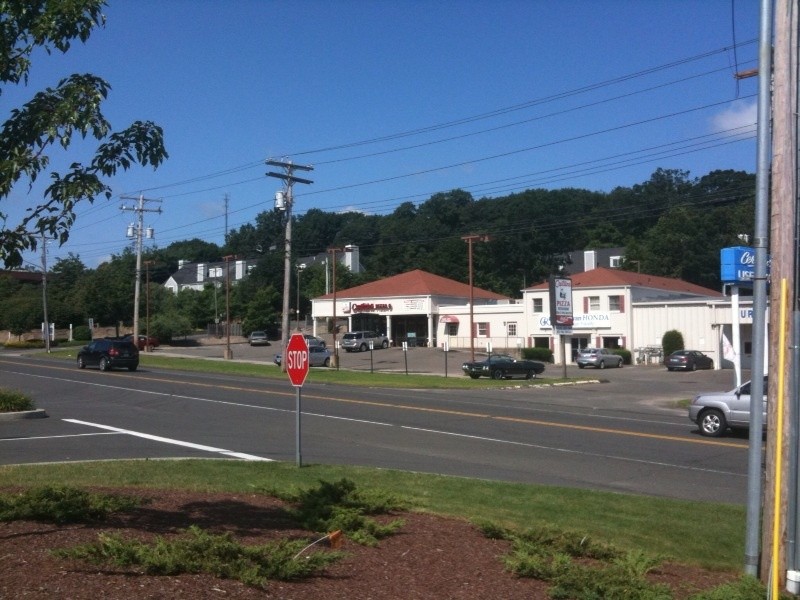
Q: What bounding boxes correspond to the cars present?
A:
[666,349,712,371]
[462,353,546,380]
[304,334,326,348]
[276,345,332,367]
[688,374,772,436]
[126,335,158,350]
[343,330,390,352]
[578,347,624,368]
[247,331,268,346]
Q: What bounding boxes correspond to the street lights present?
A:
[327,248,354,368]
[144,259,154,350]
[222,254,236,360]
[460,234,492,363]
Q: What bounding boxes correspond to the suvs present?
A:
[76,337,139,371]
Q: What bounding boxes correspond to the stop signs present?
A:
[286,333,310,387]
